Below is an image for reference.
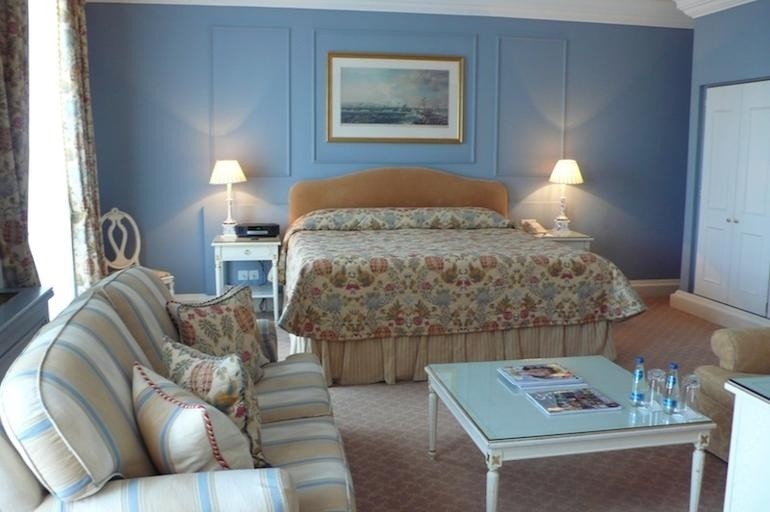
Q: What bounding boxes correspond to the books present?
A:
[497,362,587,390]
[525,383,623,416]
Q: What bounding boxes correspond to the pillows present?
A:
[131,362,255,473]
[166,282,244,342]
[176,285,271,369]
[159,334,272,467]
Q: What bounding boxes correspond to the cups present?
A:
[680,374,701,417]
[643,368,666,411]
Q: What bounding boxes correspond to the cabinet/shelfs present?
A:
[720,374,770,512]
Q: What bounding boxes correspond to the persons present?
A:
[510,367,558,382]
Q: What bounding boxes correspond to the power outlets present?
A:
[250,270,258,280]
[238,270,248,280]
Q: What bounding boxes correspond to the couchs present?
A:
[687,325,770,467]
[0,260,361,512]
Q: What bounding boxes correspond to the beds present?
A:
[279,165,645,386]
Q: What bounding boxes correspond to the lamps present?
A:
[549,159,585,233]
[208,158,247,238]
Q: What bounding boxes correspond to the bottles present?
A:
[628,356,649,409]
[661,362,682,416]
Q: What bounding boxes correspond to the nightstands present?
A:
[542,228,594,251]
[212,233,281,324]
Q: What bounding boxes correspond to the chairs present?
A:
[101,207,141,274]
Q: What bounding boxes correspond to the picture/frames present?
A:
[324,51,466,145]
